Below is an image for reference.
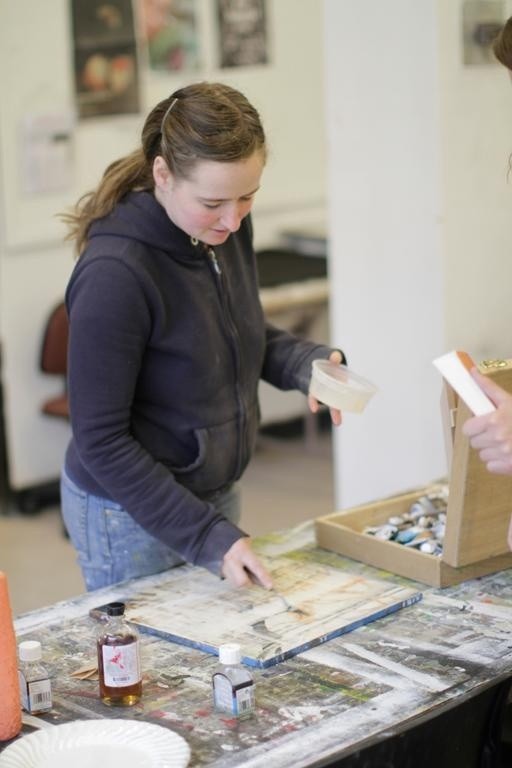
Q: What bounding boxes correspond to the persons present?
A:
[53,78,365,594]
[459,16,512,547]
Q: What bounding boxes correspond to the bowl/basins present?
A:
[307,356,376,413]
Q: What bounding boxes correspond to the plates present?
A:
[1,719,191,768]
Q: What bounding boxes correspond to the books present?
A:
[430,350,497,417]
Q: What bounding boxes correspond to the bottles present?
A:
[212,643,258,718]
[17,640,53,715]
[94,602,143,707]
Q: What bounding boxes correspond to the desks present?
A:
[0,520,512,766]
